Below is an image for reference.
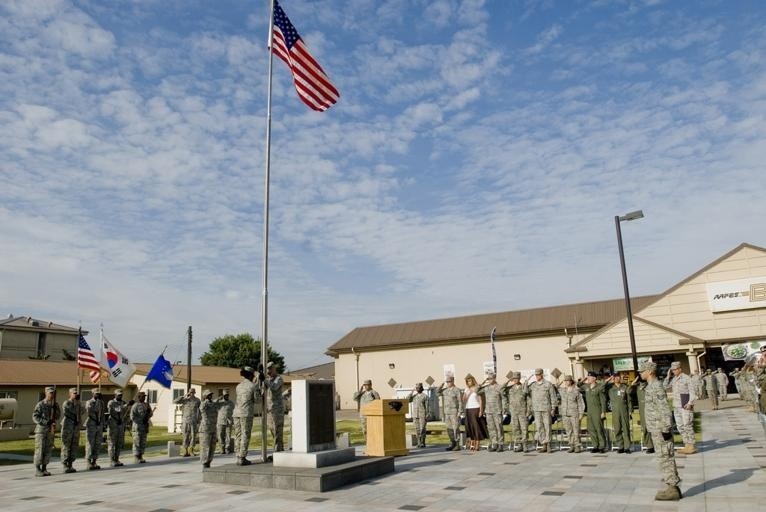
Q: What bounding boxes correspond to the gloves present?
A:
[258,370,265,381]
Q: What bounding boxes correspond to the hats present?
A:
[189,388,197,394]
[564,374,573,382]
[69,388,78,394]
[45,386,55,394]
[363,380,372,386]
[535,368,544,376]
[265,361,275,373]
[245,366,254,374]
[203,390,214,400]
[487,373,496,379]
[223,390,231,398]
[91,388,102,394]
[637,361,657,371]
[761,345,766,352]
[138,392,147,397]
[115,388,125,396]
[588,371,597,377]
[415,383,423,388]
[512,372,521,379]
[670,361,680,370]
[445,376,454,382]
[612,371,620,376]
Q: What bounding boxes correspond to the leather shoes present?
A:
[618,449,625,453]
[591,448,601,453]
[646,448,654,453]
[600,449,606,454]
[625,449,631,453]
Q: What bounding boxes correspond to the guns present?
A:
[50,384,56,432]
[143,407,156,425]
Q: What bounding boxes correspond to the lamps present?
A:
[388,364,395,370]
[514,354,521,360]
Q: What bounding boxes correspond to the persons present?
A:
[406,382,429,447]
[625,375,652,453]
[231,366,262,465]
[598,373,632,453]
[523,369,556,453]
[215,389,234,453]
[199,390,218,470]
[173,388,201,456]
[61,388,83,472]
[436,375,461,450]
[476,373,506,451]
[261,362,284,460]
[711,368,727,401]
[692,369,702,400]
[130,392,152,463]
[462,375,483,450]
[662,362,695,453]
[107,389,134,466]
[353,380,380,454]
[638,361,681,499]
[699,368,718,409]
[576,372,605,452]
[729,345,765,415]
[85,388,105,470]
[499,372,528,451]
[32,386,60,477]
[554,376,584,453]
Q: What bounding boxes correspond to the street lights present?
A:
[615,210,644,386]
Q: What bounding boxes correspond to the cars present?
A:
[578,379,639,412]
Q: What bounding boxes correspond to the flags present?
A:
[145,345,173,389]
[77,329,100,383]
[262,1,341,113]
[99,326,136,388]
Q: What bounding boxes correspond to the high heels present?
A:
[470,442,475,451]
[476,444,480,451]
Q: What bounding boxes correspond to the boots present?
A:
[523,444,528,452]
[87,462,94,472]
[92,461,101,470]
[575,446,582,453]
[236,458,239,464]
[488,447,496,451]
[567,446,575,454]
[268,455,274,462]
[515,444,524,452]
[68,463,76,473]
[225,447,232,455]
[238,458,250,464]
[116,460,124,467]
[445,441,456,451]
[110,460,119,467]
[416,437,427,448]
[547,445,551,452]
[41,465,52,476]
[496,446,503,452]
[220,447,227,455]
[678,443,696,453]
[182,449,189,458]
[188,447,196,458]
[656,486,684,501]
[62,464,70,474]
[539,445,547,452]
[134,455,147,464]
[452,442,461,451]
[34,465,46,478]
